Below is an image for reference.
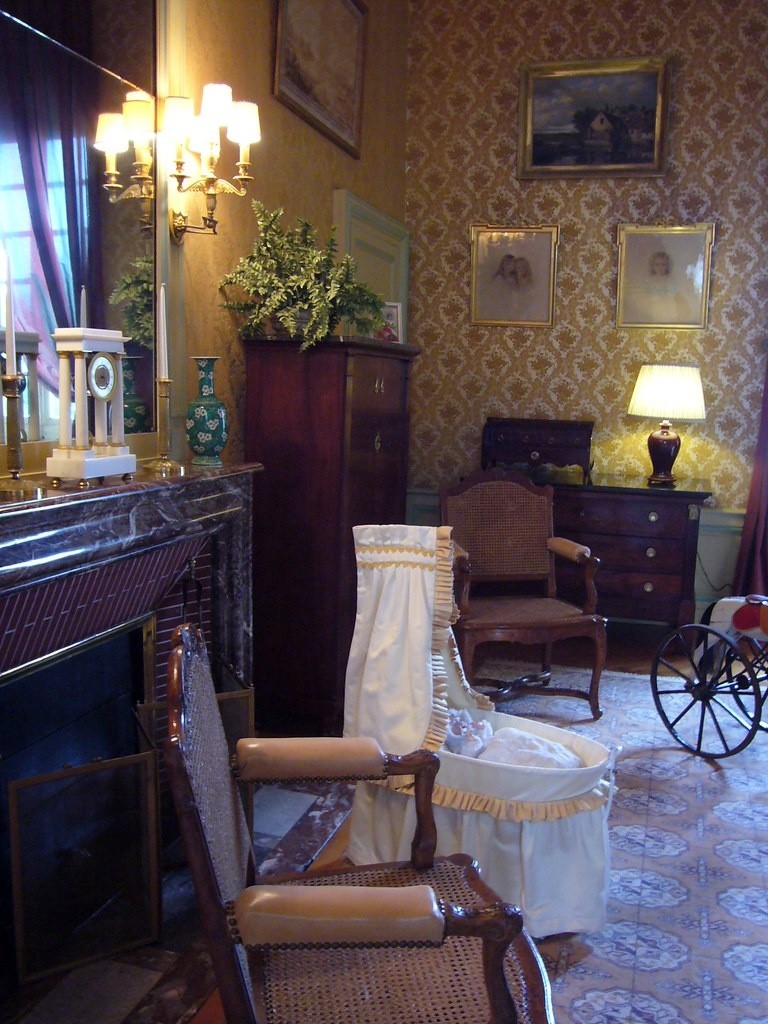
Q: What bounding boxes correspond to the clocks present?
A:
[87,352,118,402]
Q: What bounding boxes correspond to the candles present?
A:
[5,254,17,375]
[160,282,169,379]
[81,285,86,328]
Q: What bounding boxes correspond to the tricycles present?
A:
[651,597,768,759]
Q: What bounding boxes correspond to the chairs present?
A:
[162,625,554,1024]
[439,469,607,720]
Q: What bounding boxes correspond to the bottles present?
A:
[185,356,229,464]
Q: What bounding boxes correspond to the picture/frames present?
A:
[370,302,403,345]
[615,218,716,332]
[469,216,561,328]
[274,0,370,159]
[517,55,670,177]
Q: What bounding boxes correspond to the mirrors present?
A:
[0,0,169,445]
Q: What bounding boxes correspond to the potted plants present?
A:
[216,199,396,354]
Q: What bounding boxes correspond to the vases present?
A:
[108,356,146,435]
[188,356,227,467]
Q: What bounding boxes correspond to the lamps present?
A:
[93,91,155,242]
[164,82,262,247]
[627,364,706,484]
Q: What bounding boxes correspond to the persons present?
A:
[492,254,535,292]
[642,253,690,323]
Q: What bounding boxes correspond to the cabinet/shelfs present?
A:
[533,483,712,656]
[239,336,424,713]
